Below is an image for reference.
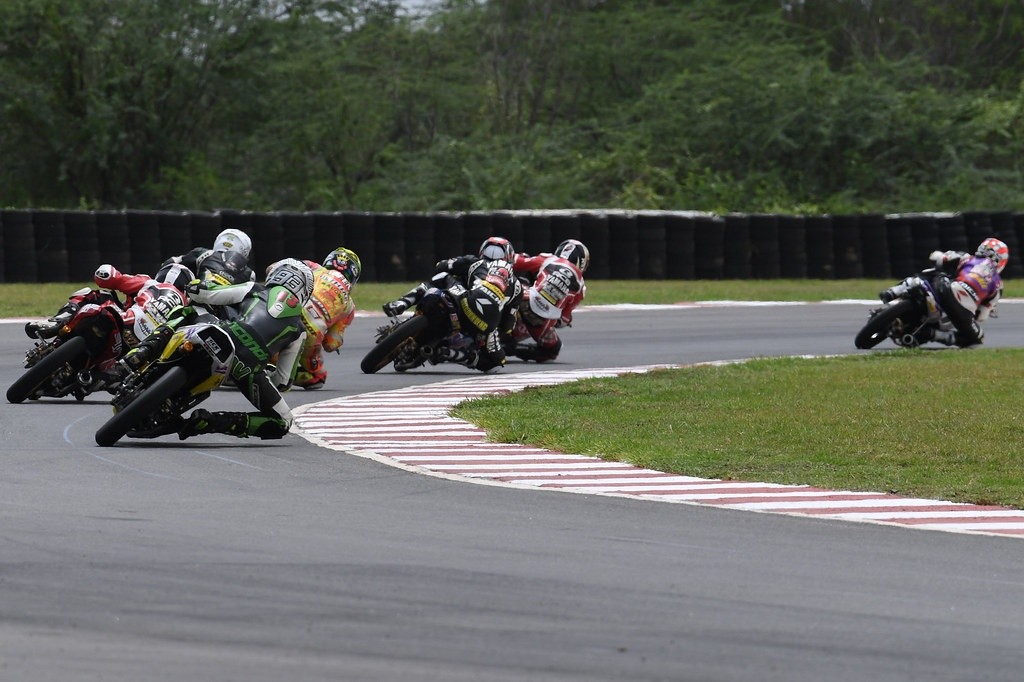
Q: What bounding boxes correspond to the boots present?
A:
[437,346,480,369]
[25,302,78,339]
[879,277,914,303]
[178,409,250,439]
[384,283,429,318]
[122,323,176,371]
[504,341,533,360]
[922,327,957,346]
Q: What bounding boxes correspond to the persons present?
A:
[381,237,590,374]
[878,237,1009,348]
[103,257,315,439]
[25,226,362,390]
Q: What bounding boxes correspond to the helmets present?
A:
[322,246,361,286]
[154,262,198,296]
[978,236,1009,273]
[555,238,589,273]
[264,257,314,307]
[479,237,515,266]
[214,229,251,259]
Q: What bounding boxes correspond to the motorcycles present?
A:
[95,321,237,447]
[5,287,125,403]
[360,285,533,375]
[852,264,958,351]
